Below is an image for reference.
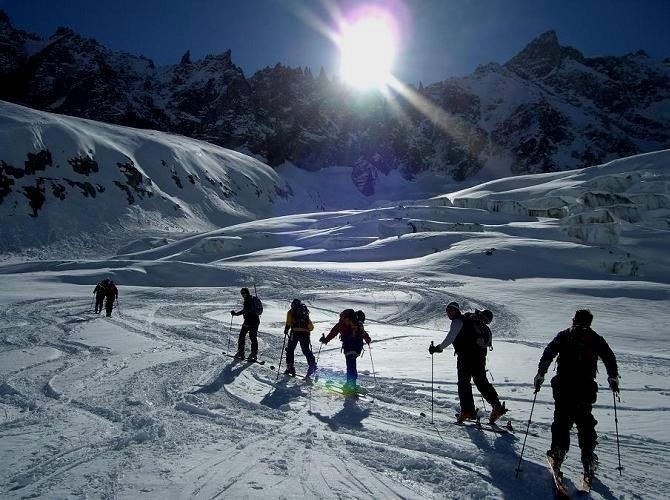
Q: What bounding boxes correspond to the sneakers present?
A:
[248,352,257,360]
[342,383,360,394]
[307,365,316,376]
[548,448,564,466]
[454,410,478,424]
[284,366,296,375]
[489,403,504,423]
[234,350,246,358]
[581,451,596,475]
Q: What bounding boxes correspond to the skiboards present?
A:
[421,413,515,434]
[270,366,313,382]
[222,352,265,364]
[327,384,368,398]
[546,451,598,500]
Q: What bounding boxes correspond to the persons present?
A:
[531,307,622,475]
[281,298,319,378]
[429,300,509,426]
[229,288,262,359]
[93,279,120,318]
[321,309,374,396]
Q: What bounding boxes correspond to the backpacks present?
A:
[251,294,263,316]
[291,303,310,328]
[463,309,493,348]
[344,309,364,337]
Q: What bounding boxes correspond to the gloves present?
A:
[284,325,291,336]
[319,334,330,345]
[231,309,239,317]
[363,332,371,346]
[607,376,621,393]
[429,344,442,356]
[533,373,545,393]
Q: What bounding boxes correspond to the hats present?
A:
[478,308,492,323]
[574,310,593,326]
[291,298,301,307]
[447,302,461,312]
[240,287,250,295]
